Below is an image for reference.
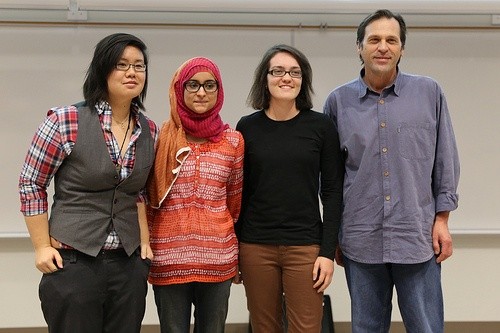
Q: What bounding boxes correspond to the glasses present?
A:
[185,80,217,92]
[268,69,303,78]
[115,62,147,71]
